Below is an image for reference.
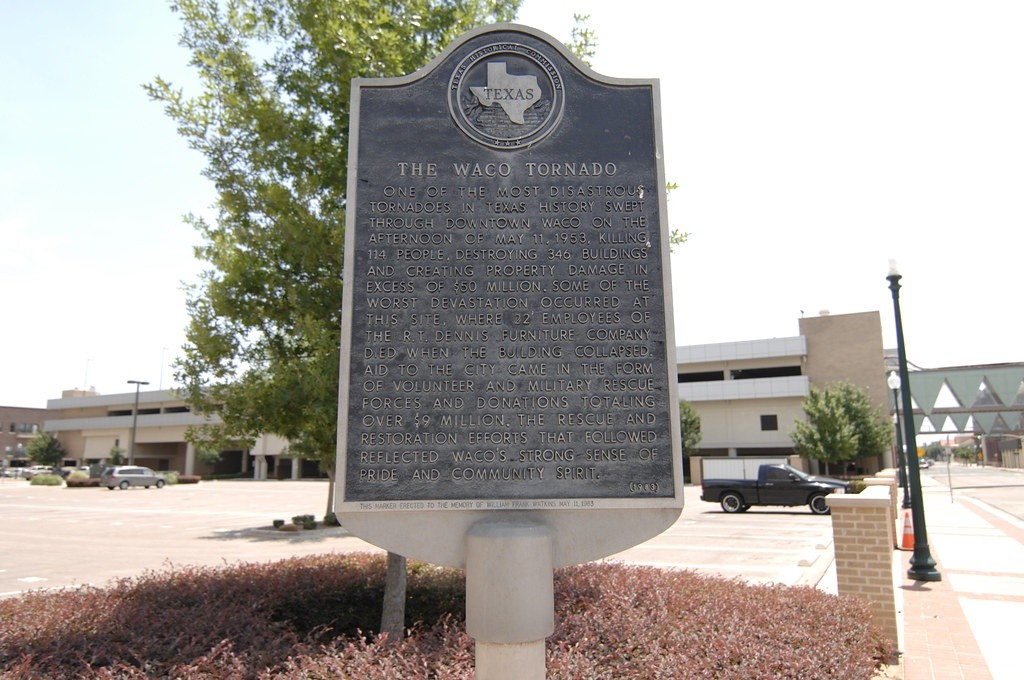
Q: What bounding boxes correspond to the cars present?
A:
[98,465,167,490]
[4,462,111,487]
[918,458,935,469]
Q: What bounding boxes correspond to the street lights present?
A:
[886,370,913,510]
[883,256,943,582]
[126,378,151,466]
[892,412,905,488]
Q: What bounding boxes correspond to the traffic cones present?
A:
[895,511,916,551]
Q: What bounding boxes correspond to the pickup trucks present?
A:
[698,463,854,516]
